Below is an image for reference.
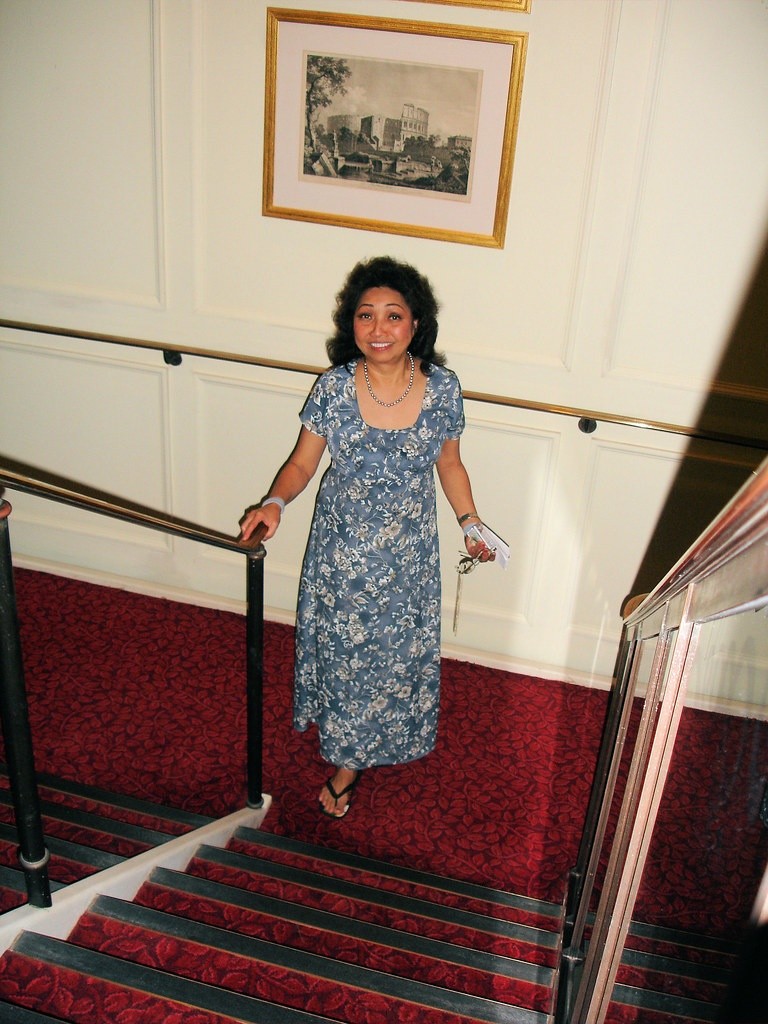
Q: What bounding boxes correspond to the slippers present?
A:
[319,769,361,819]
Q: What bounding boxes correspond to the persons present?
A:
[239,257,496,821]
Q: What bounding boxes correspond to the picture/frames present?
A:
[260,6,531,250]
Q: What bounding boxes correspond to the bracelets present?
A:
[262,497,285,514]
[458,512,479,526]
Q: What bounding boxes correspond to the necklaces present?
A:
[363,352,414,407]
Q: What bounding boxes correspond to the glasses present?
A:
[455,547,497,574]
